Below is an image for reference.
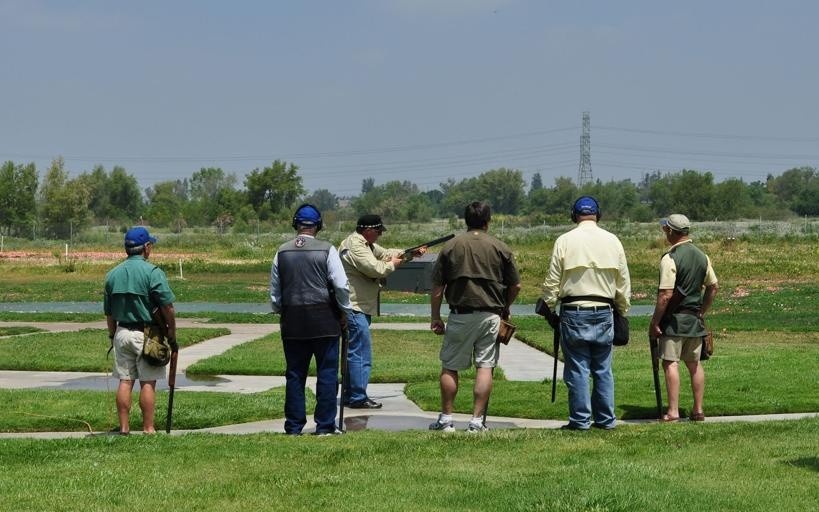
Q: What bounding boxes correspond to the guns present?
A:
[536,298,559,402]
[399,233,455,258]
[328,278,349,433]
[152,307,178,434]
[648,285,686,422]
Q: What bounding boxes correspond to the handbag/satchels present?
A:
[613,310,629,345]
[499,320,516,345]
[143,324,170,366]
[700,328,713,360]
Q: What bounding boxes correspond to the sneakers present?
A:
[429,414,455,431]
[465,422,490,432]
[690,411,704,423]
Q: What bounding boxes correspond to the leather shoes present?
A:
[344,398,382,408]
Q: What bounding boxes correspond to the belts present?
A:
[564,306,609,310]
[451,308,494,314]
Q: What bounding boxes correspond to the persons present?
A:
[269,204,353,437]
[541,196,631,432]
[429,202,522,434]
[649,213,720,422]
[337,214,428,409]
[104,226,177,437]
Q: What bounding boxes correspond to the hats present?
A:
[357,215,386,231]
[575,197,597,215]
[125,227,156,247]
[295,206,321,224]
[667,214,690,233]
